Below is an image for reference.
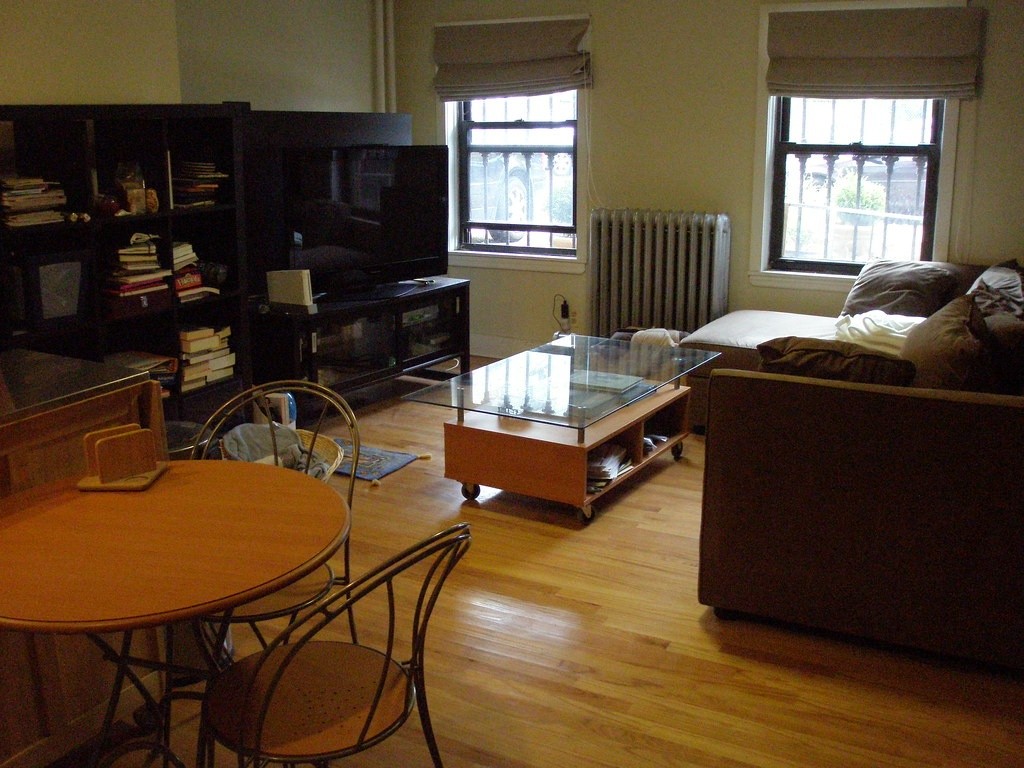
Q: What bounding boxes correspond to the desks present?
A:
[1,458,351,767]
[254,276,478,419]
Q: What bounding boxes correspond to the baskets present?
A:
[222,428,344,484]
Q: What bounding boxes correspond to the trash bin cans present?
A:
[164,419,217,461]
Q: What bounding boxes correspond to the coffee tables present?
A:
[400,332,723,525]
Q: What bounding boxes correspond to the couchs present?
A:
[679,257,1024,664]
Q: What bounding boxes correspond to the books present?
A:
[252,391,290,428]
[265,267,318,355]
[531,368,659,421]
[0,159,237,397]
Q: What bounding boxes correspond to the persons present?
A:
[260,397,279,420]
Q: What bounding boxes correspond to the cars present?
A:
[470,151,544,243]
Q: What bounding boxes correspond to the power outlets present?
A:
[561,316,570,331]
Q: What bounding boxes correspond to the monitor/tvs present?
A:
[244,145,449,303]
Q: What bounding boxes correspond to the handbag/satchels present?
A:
[253,399,297,432]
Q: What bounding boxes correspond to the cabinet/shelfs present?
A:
[0,103,253,429]
[0,371,186,768]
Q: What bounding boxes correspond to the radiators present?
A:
[589,206,731,338]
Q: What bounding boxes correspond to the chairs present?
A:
[198,519,473,765]
[157,379,362,764]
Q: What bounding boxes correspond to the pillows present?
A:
[756,335,916,387]
[984,310,1024,396]
[901,289,989,391]
